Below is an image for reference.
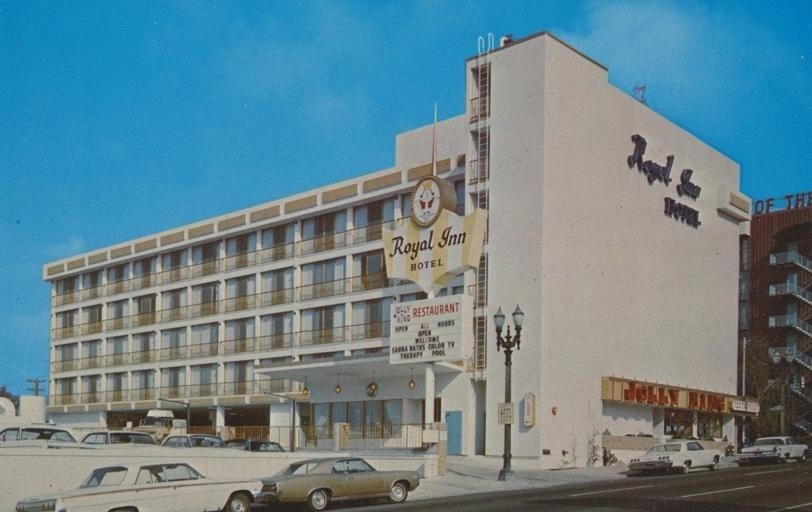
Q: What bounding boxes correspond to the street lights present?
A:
[493,304,524,482]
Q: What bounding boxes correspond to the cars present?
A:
[0,424,286,453]
[625,439,724,477]
[262,456,420,511]
[16,461,263,512]
[738,435,809,467]
